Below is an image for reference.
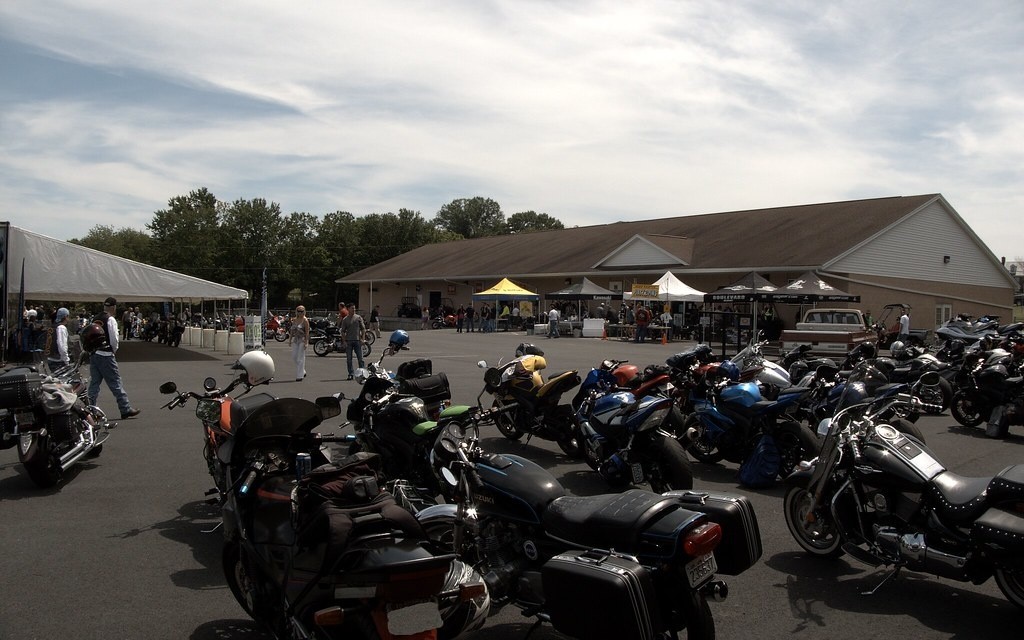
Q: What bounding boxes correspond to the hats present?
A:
[55,307,69,322]
[104,297,118,306]
[601,302,605,305]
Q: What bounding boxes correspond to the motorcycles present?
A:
[158,312,1024,638]
[78,318,90,335]
[0,347,119,487]
[432,314,459,330]
[131,316,145,338]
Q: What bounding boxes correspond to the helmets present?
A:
[951,340,965,353]
[390,329,410,347]
[438,558,491,640]
[238,350,275,386]
[789,361,808,383]
[890,341,903,357]
[984,348,1011,365]
[860,342,874,359]
[81,324,105,350]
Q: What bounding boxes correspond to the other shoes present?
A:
[347,375,353,381]
[121,408,141,419]
[633,338,645,343]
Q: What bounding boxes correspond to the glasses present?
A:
[298,310,304,313]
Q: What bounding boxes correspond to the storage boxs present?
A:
[50,409,82,440]
[230,392,280,433]
[0,368,43,409]
[0,412,19,450]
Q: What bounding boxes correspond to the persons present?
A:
[438,304,445,317]
[338,302,350,326]
[88,297,140,419]
[341,303,366,380]
[23,303,87,355]
[421,306,429,330]
[595,300,652,343]
[543,305,561,338]
[865,309,873,327]
[897,309,909,341]
[711,303,779,322]
[370,305,382,337]
[46,308,70,372]
[795,307,806,324]
[288,305,309,381]
[456,302,519,333]
[121,306,188,347]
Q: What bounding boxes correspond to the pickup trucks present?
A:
[777,308,879,361]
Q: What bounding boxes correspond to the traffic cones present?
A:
[601,328,608,340]
[660,334,669,344]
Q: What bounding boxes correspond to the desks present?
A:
[547,321,583,335]
[609,325,671,340]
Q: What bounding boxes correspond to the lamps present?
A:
[564,278,572,285]
[944,255,951,264]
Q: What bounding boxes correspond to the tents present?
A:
[704,271,780,345]
[472,278,540,332]
[0,222,249,354]
[543,276,623,324]
[769,269,860,324]
[623,271,714,340]
